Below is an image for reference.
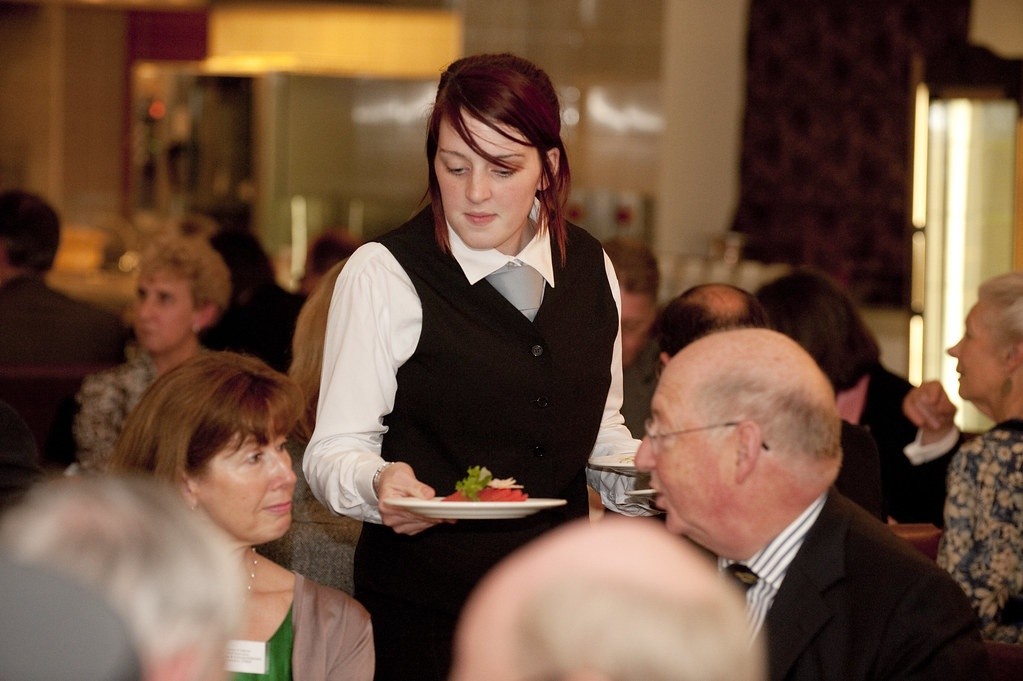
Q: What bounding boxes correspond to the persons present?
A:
[601,236,940,529]
[902,268,1023,650]
[301,50,640,681]
[0,189,378,681]
[633,328,994,681]
[455,513,766,681]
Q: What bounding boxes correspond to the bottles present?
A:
[644,195,656,245]
[567,207,582,227]
[616,191,635,237]
[722,221,746,278]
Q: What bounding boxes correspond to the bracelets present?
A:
[373,468,381,497]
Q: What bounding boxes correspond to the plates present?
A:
[589,451,637,470]
[624,489,658,496]
[384,497,567,519]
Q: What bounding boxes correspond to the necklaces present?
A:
[248,548,258,589]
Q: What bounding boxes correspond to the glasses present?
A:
[644,416,769,456]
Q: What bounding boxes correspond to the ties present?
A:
[724,562,757,604]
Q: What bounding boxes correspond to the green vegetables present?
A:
[455,465,493,502]
